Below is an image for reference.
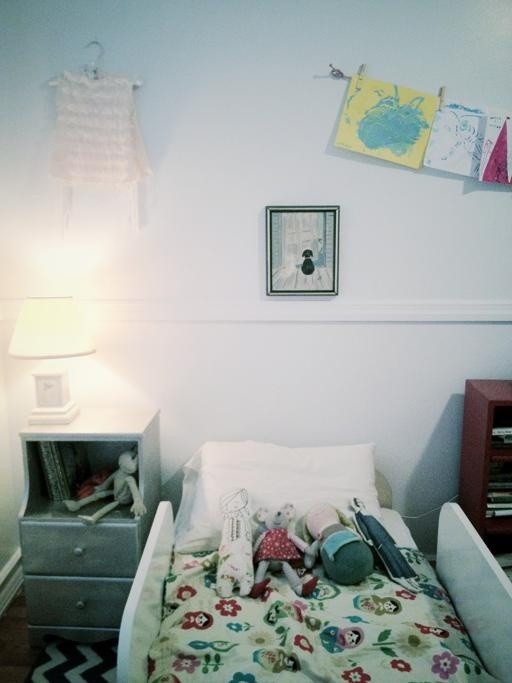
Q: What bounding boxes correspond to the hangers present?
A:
[48,40,143,88]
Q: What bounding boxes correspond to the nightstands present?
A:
[18,408,161,649]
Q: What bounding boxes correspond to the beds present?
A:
[116,467,511,683]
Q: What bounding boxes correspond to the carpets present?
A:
[23,638,119,683]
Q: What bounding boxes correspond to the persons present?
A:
[353,497,419,587]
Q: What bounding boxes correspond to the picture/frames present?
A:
[264,205,339,296]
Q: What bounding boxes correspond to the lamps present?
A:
[9,297,98,427]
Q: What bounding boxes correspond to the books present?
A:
[485,427,512,519]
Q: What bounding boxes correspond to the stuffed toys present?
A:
[250,505,319,598]
[62,450,147,525]
[301,502,374,585]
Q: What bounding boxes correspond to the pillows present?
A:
[173,439,384,553]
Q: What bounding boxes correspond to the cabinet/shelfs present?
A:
[458,380,511,555]
